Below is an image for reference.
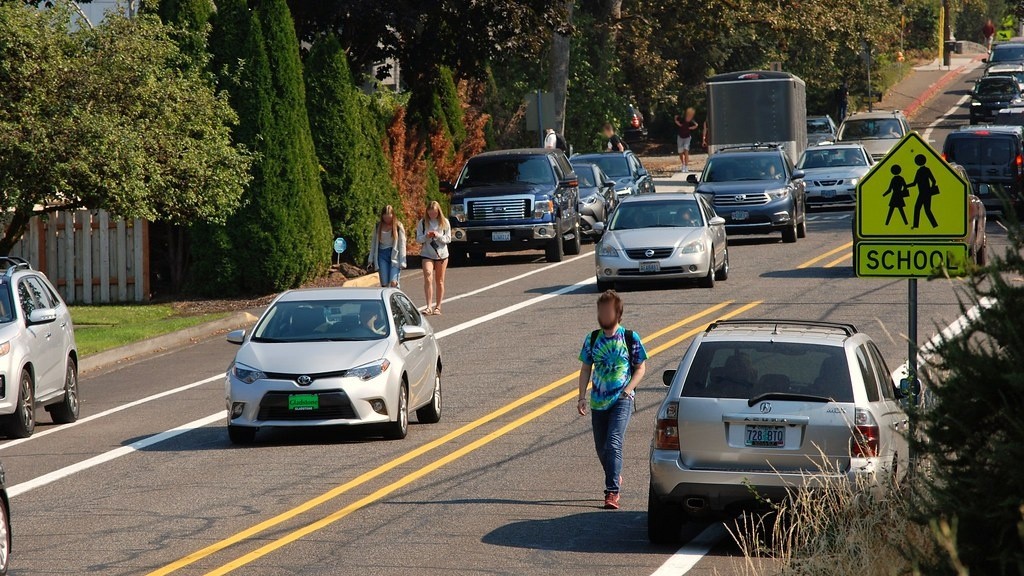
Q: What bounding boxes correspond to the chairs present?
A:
[675,206,699,226]
[610,160,626,174]
[704,366,729,390]
[759,374,790,395]
[292,305,314,330]
[819,356,843,378]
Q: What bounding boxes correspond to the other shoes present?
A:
[681,164,689,173]
[422,308,441,316]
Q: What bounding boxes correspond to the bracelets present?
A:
[578,397,586,403]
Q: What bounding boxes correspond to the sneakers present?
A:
[604,476,622,509]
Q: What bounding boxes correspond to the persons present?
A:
[604,123,624,152]
[880,124,900,138]
[839,152,863,165]
[576,290,649,510]
[417,200,451,314]
[368,204,407,289]
[543,129,556,149]
[352,302,387,336]
[676,207,701,227]
[758,162,781,180]
[675,107,708,173]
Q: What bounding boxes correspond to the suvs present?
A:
[567,150,655,204]
[939,122,1024,223]
[947,161,987,269]
[0,256,80,438]
[685,141,807,245]
[647,318,923,540]
[826,109,911,165]
[439,148,582,264]
[967,40,1024,126]
[806,114,839,147]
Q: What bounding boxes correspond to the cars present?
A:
[795,144,876,213]
[570,163,619,244]
[224,287,442,446]
[0,460,12,576]
[592,192,730,293]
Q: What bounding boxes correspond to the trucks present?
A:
[706,70,808,171]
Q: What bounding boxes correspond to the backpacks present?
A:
[546,133,567,152]
[620,139,630,151]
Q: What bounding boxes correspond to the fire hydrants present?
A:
[896,52,905,62]
